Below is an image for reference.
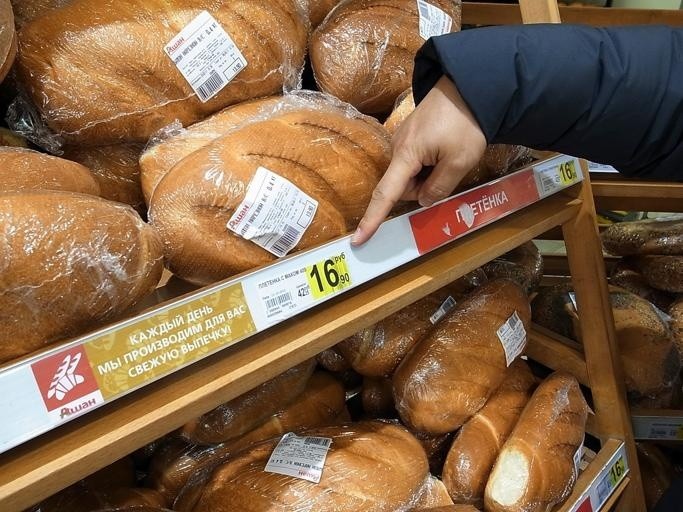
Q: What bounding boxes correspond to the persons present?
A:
[350,23,683,248]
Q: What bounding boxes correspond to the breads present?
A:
[1,1,683,512]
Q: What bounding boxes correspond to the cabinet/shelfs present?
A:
[0,0,683,512]
